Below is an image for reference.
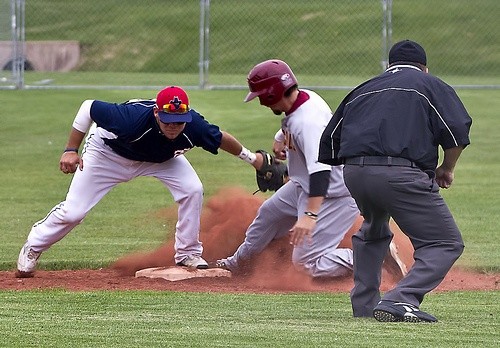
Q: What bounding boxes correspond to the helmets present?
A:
[244,59,297,102]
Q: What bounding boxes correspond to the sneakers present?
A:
[383,240,408,283]
[17,241,42,272]
[209,261,249,279]
[372,300,437,322]
[178,255,209,269]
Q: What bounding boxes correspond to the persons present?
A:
[203,60,408,285]
[319,39,470,322]
[18,84,286,279]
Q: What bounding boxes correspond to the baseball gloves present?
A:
[254,149,290,192]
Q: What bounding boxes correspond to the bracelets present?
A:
[303,211,319,221]
[61,147,79,155]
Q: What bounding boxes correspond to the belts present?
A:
[345,156,418,168]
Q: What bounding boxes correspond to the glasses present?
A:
[160,104,189,115]
[159,119,185,125]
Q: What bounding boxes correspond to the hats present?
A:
[156,86,192,122]
[389,40,427,66]
